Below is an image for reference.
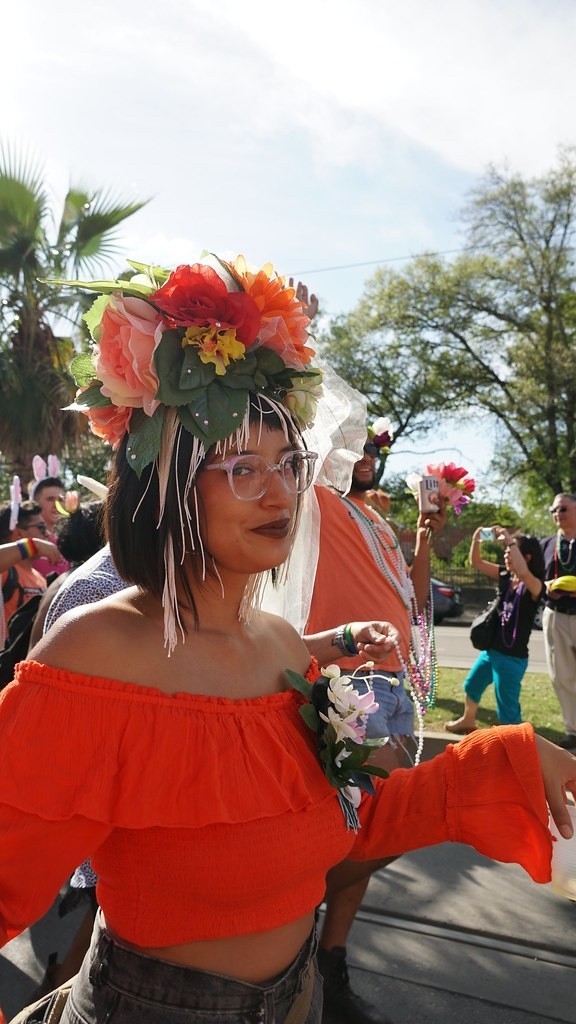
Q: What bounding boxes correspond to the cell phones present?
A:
[479,528,496,541]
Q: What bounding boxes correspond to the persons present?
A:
[251,278,447,772]
[539,491,576,749]
[445,525,545,731]
[0,251,576,1024]
[0,455,129,690]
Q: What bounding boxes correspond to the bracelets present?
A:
[509,543,515,546]
[335,622,359,658]
[16,537,38,560]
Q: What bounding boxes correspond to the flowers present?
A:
[414,461,476,544]
[32,250,326,482]
[283,661,399,836]
[363,416,396,458]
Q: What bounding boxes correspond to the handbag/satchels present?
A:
[470,595,504,650]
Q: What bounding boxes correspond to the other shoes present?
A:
[445,719,477,733]
[559,733,576,748]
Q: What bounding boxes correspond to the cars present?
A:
[429,580,464,625]
[533,599,547,630]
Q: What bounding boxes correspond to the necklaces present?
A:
[501,581,523,648]
[333,490,416,608]
[363,636,424,766]
[554,529,576,579]
[408,581,439,716]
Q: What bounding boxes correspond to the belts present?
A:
[547,604,576,614]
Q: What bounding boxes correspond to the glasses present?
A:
[200,450,317,501]
[364,444,380,458]
[21,522,45,534]
[549,506,576,513]
